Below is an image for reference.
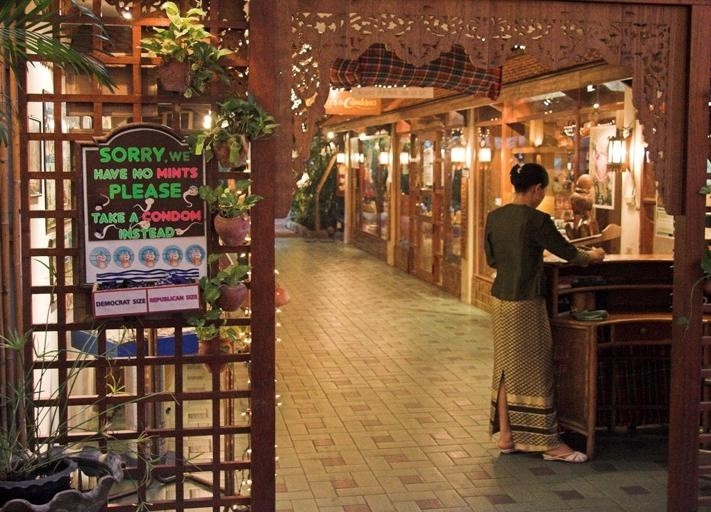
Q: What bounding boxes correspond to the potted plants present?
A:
[0,317,126,508]
[135,1,235,94]
[189,100,282,376]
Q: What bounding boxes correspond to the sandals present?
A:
[542,449,589,464]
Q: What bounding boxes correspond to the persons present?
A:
[564,190,601,256]
[484,162,608,463]
